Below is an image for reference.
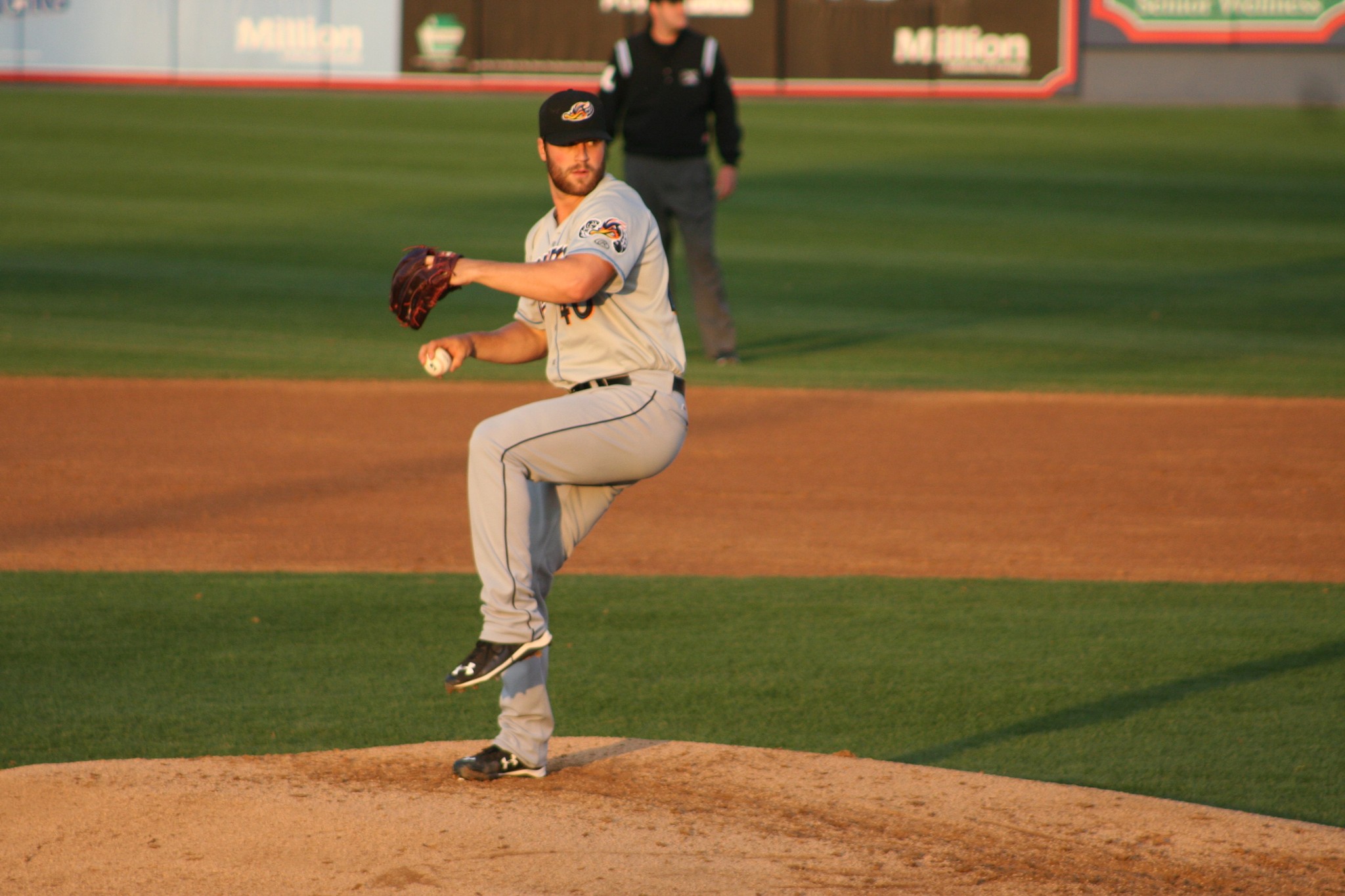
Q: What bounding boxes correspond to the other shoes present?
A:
[715,352,740,365]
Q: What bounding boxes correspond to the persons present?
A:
[594,0,744,367]
[387,90,690,782]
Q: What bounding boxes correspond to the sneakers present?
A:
[445,630,552,688]
[453,745,546,780]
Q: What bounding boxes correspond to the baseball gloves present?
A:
[388,244,464,331]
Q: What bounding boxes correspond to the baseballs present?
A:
[421,347,452,377]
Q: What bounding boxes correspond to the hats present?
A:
[538,89,611,145]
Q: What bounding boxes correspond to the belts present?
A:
[570,376,685,393]
